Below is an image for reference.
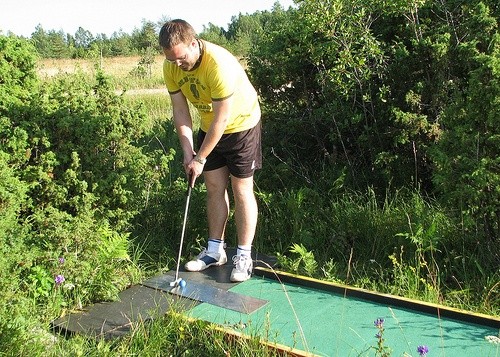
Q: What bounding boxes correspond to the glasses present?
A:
[165,42,193,63]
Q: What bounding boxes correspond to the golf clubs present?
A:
[169,169,201,288]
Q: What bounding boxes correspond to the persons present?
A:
[159,19,262,281]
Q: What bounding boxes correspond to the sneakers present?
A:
[229,252,253,282]
[184,247,228,272]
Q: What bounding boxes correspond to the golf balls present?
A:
[179,280,187,287]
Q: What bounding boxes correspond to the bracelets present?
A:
[194,155,206,164]
